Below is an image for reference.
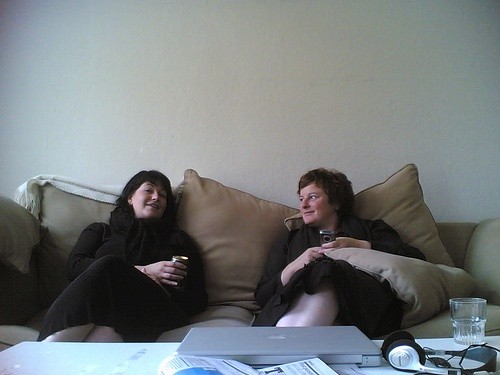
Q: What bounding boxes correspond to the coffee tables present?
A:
[0,335,500,375]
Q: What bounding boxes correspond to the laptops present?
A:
[176,326,382,369]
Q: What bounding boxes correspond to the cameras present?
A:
[320,230,336,246]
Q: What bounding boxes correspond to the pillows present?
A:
[320,245,473,328]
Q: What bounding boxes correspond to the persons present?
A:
[36,170,206,343]
[251,168,426,338]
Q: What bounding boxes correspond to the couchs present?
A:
[1,174,500,351]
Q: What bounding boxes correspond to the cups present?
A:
[449,297,488,346]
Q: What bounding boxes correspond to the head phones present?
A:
[380,330,497,375]
